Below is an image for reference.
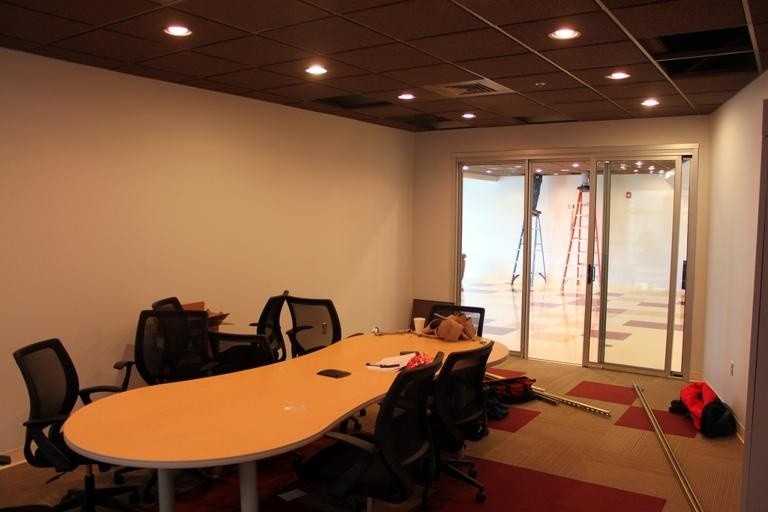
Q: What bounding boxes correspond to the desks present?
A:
[63,331,510,512]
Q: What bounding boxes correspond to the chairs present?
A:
[350,341,494,503]
[13,339,137,512]
[378,305,485,447]
[298,352,444,511]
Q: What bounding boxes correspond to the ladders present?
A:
[560,190,601,296]
[511,214,547,285]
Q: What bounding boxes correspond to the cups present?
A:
[413,318,426,333]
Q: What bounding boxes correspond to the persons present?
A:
[532,172,542,215]
[576,170,590,189]
